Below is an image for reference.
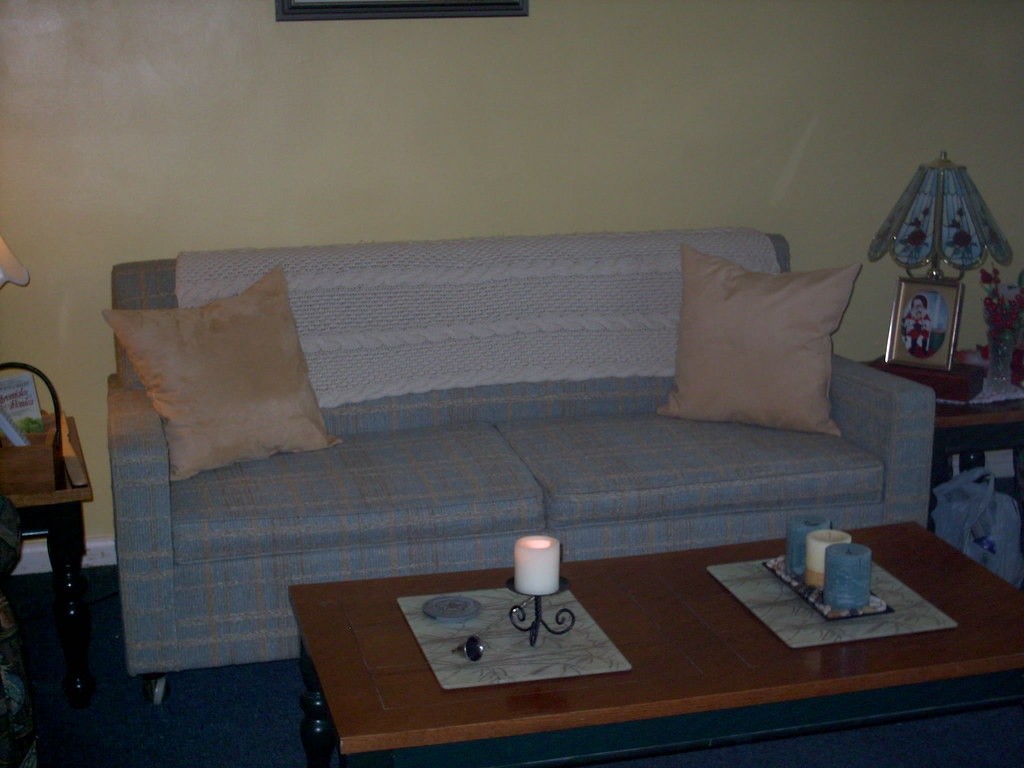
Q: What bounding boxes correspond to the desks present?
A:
[0,416,95,709]
[927,399,1024,531]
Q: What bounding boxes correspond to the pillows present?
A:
[100,267,346,484]
[654,243,863,436]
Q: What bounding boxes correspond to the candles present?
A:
[824,542,872,609]
[804,529,852,589]
[784,513,830,574]
[514,535,561,596]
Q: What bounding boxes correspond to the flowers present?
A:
[978,260,1024,337]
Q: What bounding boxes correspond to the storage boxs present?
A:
[874,355,983,401]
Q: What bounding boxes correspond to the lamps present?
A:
[864,151,1014,401]
[0,235,33,449]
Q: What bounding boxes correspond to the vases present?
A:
[983,334,1015,399]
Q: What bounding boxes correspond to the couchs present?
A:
[102,226,938,712]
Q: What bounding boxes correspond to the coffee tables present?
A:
[286,520,1024,768]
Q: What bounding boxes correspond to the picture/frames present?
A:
[879,274,965,374]
